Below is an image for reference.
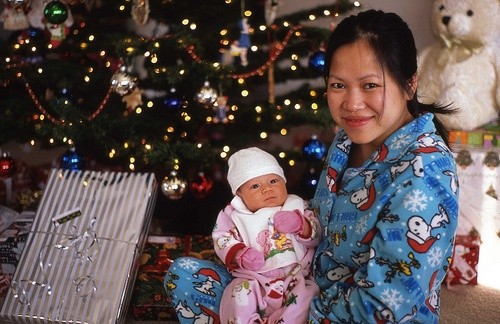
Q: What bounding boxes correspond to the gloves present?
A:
[241,247,265,271]
[273,210,301,233]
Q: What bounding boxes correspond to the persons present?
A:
[164,10,459,324]
[212,146,323,324]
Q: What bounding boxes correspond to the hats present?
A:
[226,147,287,197]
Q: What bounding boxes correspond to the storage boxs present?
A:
[0,168,157,324]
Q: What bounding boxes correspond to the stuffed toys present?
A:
[417,0,500,130]
